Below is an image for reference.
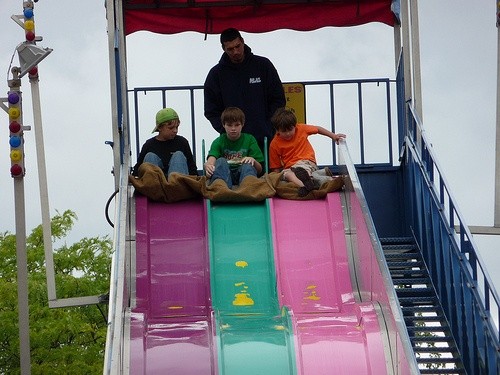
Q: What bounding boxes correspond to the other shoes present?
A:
[294,167,315,190]
[298,186,308,197]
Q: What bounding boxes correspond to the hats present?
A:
[152,108,179,133]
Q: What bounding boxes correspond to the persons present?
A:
[203,107,264,191]
[269,108,346,196]
[204,28,285,152]
[131,108,199,183]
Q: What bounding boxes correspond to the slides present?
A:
[206,197,297,375]
[270,192,395,375]
[123,184,217,375]
